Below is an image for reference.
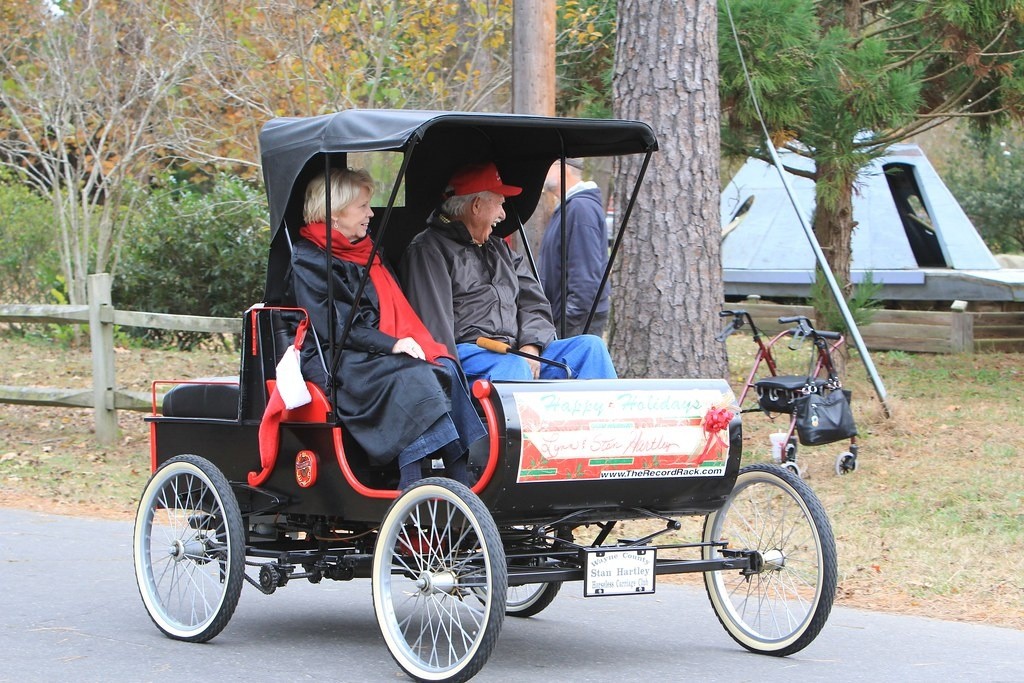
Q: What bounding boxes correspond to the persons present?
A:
[533,158,612,336]
[275,169,488,488]
[399,161,618,379]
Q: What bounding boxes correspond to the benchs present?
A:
[250,301,487,428]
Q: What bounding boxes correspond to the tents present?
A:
[719,133,1003,270]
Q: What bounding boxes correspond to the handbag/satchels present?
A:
[789,336,857,445]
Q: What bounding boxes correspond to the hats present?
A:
[558,157,585,170]
[442,161,523,197]
